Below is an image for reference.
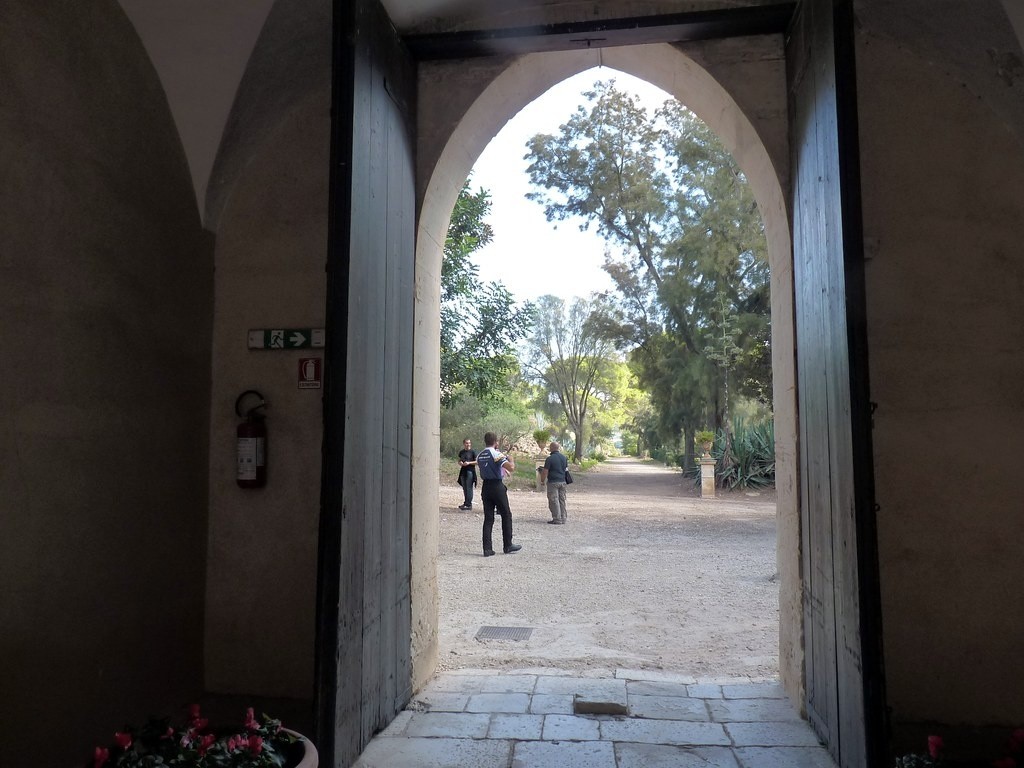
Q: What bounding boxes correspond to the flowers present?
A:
[93,703,308,768]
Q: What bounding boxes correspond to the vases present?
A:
[280,727,323,768]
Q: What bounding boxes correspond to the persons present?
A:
[477,432,522,557]
[458,438,478,510]
[541,442,568,524]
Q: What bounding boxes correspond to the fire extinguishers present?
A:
[234,390,274,489]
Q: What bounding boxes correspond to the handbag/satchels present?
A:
[565,471,573,485]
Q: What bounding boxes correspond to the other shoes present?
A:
[484,550,496,557]
[547,521,562,525]
[459,505,472,510]
[503,544,522,553]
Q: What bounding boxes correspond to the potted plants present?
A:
[533,429,551,457]
[695,431,715,458]
